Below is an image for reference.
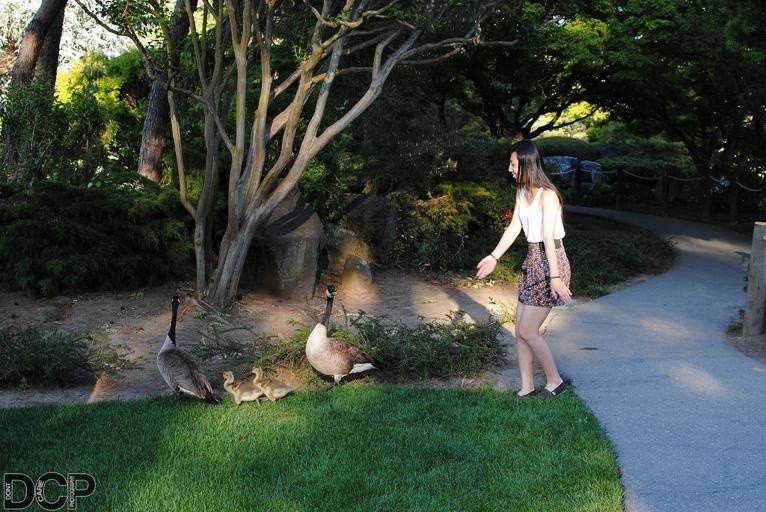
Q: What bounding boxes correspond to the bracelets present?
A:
[549,276,561,280]
[489,253,499,262]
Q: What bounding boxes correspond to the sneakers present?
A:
[515,387,537,397]
[538,380,567,398]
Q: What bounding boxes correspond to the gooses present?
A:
[156,295,221,406]
[249,365,295,405]
[304,284,385,387]
[222,369,263,411]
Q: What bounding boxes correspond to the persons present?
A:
[473,139,574,399]
